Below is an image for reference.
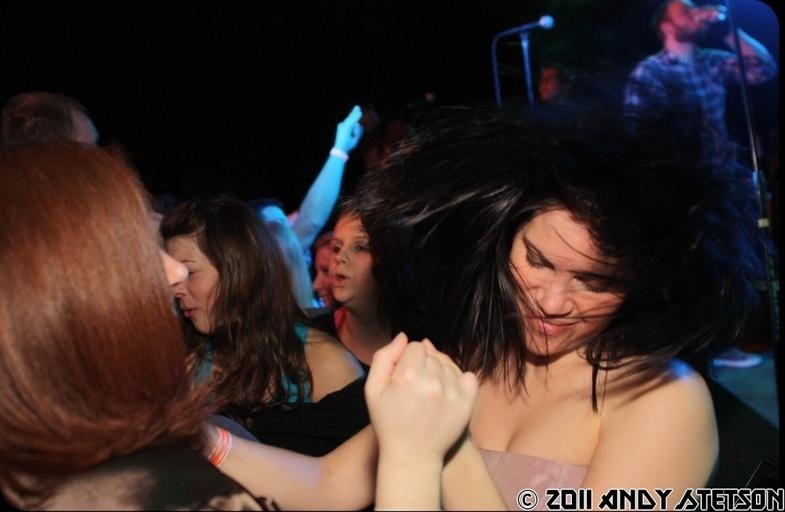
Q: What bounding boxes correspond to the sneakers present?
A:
[713,349,761,368]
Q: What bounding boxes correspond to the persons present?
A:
[2,85,721,512]
[622,0,778,343]
[536,61,581,107]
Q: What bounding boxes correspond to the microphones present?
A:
[699,7,733,36]
[497,15,554,43]
